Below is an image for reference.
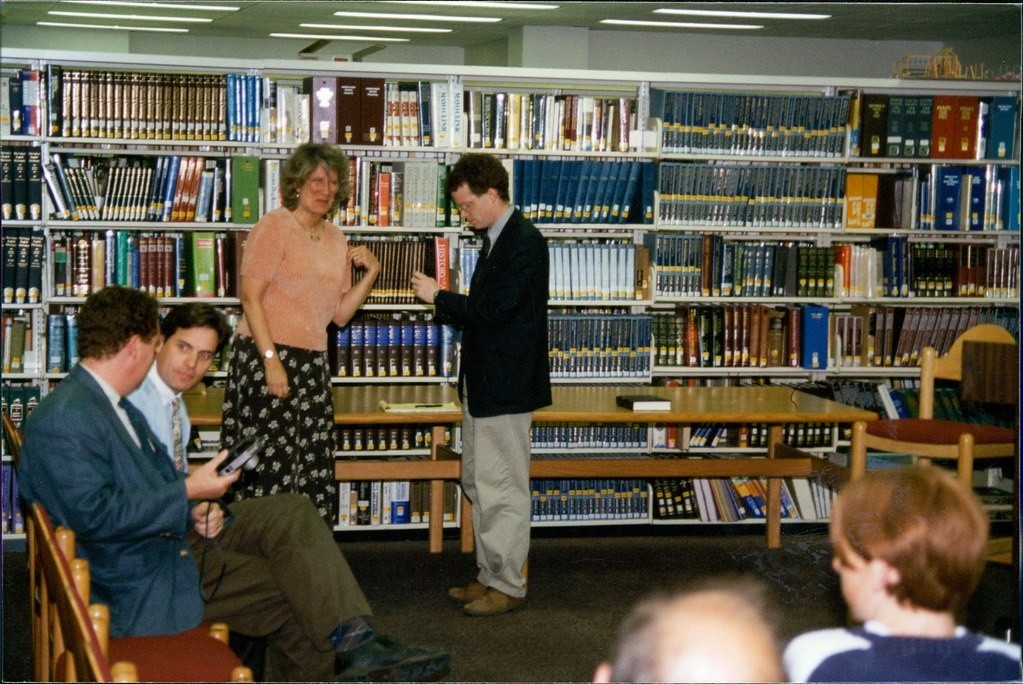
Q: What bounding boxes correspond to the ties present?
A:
[118,398,158,467]
[172,398,184,472]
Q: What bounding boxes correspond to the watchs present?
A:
[262,350,276,359]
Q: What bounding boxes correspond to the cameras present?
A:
[215,435,261,476]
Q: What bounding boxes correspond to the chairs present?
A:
[862,324,1018,564]
[0,410,254,684]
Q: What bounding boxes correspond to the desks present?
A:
[180,386,879,554]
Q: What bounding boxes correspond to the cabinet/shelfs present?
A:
[0,48,1023,531]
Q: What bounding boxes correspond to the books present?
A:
[0,64,1021,526]
[616,395,671,410]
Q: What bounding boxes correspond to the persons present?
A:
[16,284,451,683]
[411,154,553,616]
[783,465,1022,683]
[219,141,383,533]
[125,302,230,473]
[593,579,785,684]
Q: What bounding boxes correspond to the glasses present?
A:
[304,178,340,194]
[455,193,486,212]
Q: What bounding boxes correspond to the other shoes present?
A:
[334,631,450,682]
[448,582,489,604]
[462,588,527,616]
